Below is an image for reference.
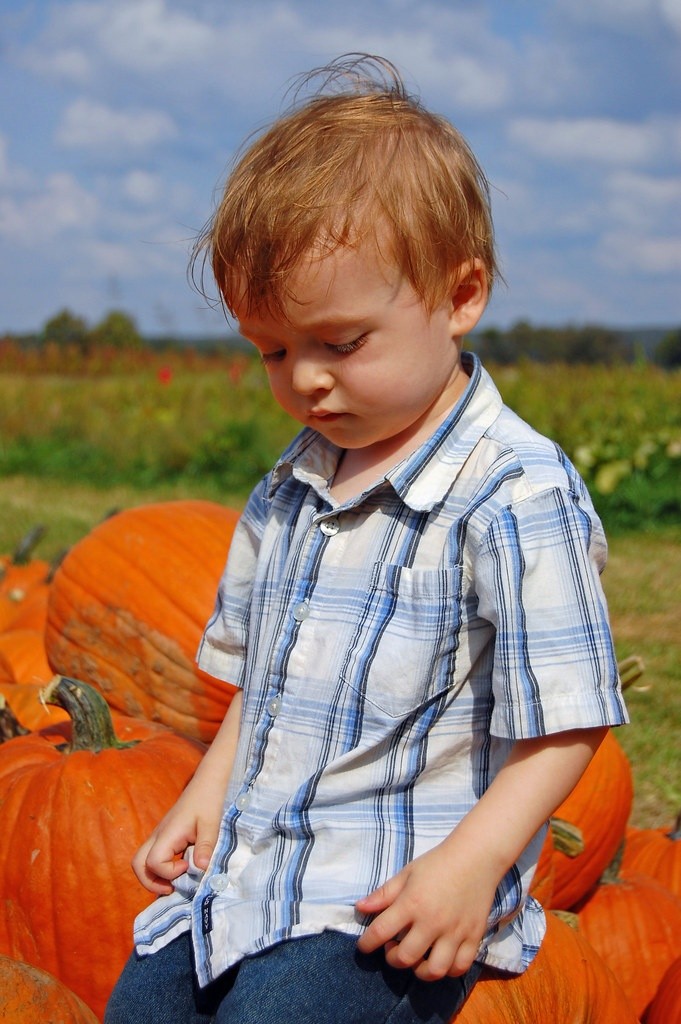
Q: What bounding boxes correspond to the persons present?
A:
[94,84,630,1024]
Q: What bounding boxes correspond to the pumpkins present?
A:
[0,501,238,1024]
[452,659,681,1024]
